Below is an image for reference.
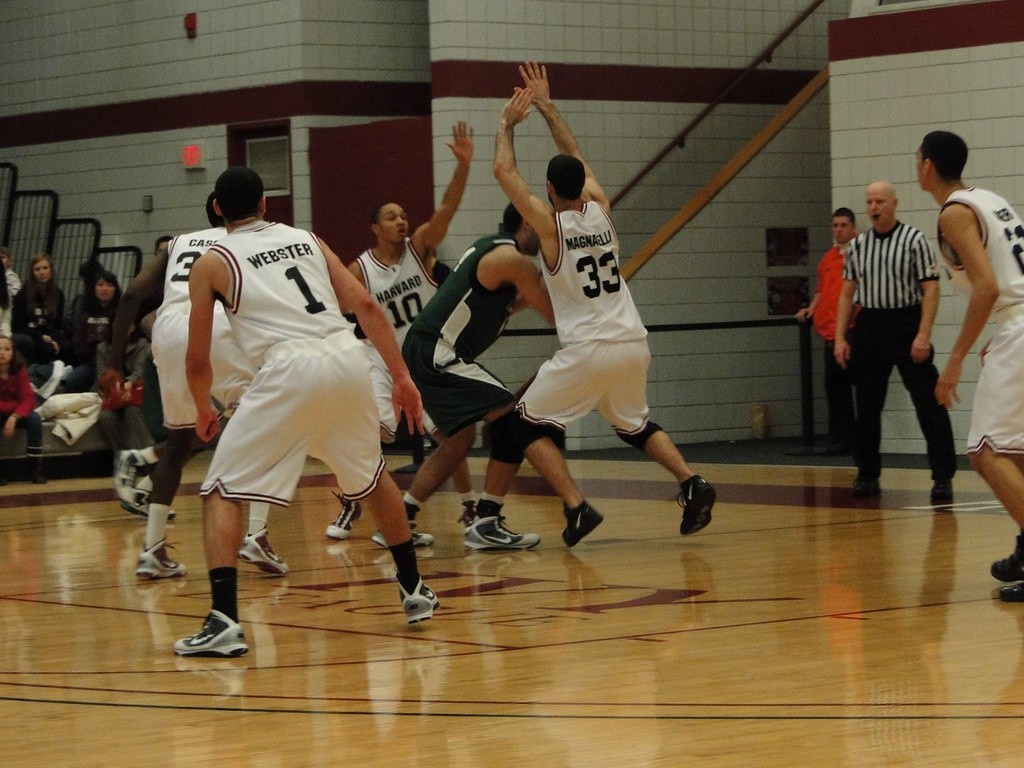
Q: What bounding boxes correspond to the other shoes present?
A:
[930,477,953,498]
[989,533,1024,603]
[29,460,48,483]
[823,442,850,457]
[854,478,880,494]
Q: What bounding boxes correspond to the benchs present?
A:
[0,415,112,459]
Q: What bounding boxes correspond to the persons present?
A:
[834,182,957,500]
[134,191,291,577]
[493,61,716,546]
[798,208,863,458]
[915,131,1024,602]
[325,120,554,550]
[173,166,439,657]
[0,236,177,519]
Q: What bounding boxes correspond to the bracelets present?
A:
[11,414,20,420]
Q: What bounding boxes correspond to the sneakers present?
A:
[324,493,364,538]
[679,551,727,633]
[459,502,480,527]
[398,575,440,625]
[327,540,367,580]
[372,519,437,549]
[182,660,248,699]
[463,514,541,554]
[467,552,542,584]
[680,476,716,536]
[563,550,608,610]
[134,539,187,581]
[372,545,434,584]
[113,446,177,520]
[174,609,249,659]
[562,500,603,548]
[137,579,190,610]
[237,528,290,577]
